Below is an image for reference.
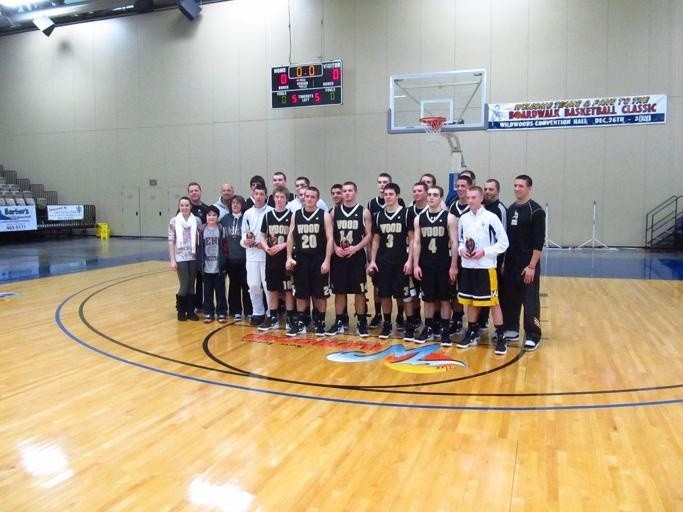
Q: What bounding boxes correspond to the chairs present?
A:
[0,175,46,210]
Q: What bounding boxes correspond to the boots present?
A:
[176,295,186,320]
[186,294,199,321]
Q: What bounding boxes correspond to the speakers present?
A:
[177,0,202,21]
[32,14,55,37]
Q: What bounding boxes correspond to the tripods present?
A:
[542,203,562,249]
[579,201,608,249]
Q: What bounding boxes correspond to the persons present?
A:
[167,166,547,358]
